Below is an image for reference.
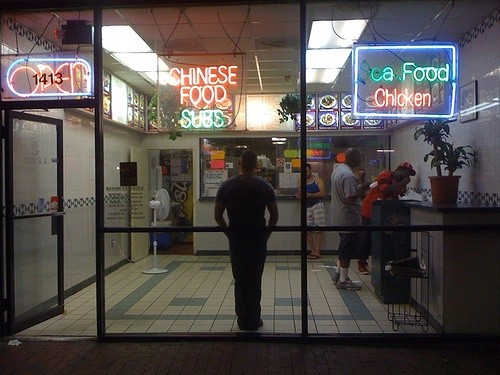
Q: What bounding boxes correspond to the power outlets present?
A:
[111,240,114,247]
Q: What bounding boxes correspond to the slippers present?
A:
[307,253,322,259]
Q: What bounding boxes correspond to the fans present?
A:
[112,188,171,274]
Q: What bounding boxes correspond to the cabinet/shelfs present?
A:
[370,200,500,334]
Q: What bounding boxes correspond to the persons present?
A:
[214,150,278,330]
[295,164,327,259]
[331,147,416,291]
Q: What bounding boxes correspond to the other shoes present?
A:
[238,319,263,330]
[359,262,369,274]
[332,271,362,290]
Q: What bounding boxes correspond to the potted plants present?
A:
[414,118,478,203]
[277,90,313,130]
[147,82,186,141]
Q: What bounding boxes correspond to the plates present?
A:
[342,95,351,108]
[298,114,314,126]
[342,113,359,126]
[319,113,335,126]
[320,95,336,108]
[365,113,381,125]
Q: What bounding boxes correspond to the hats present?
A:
[398,162,416,176]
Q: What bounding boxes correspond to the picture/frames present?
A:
[459,80,478,123]
